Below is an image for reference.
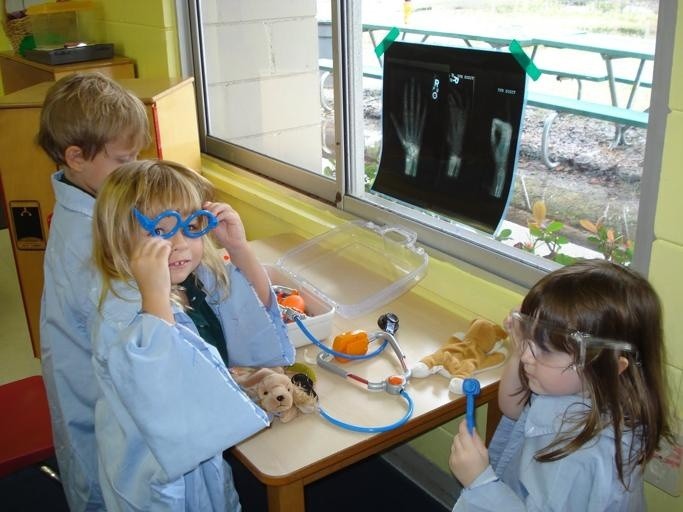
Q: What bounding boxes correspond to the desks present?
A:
[215,232,512,512]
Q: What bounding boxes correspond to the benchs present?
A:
[318,19,654,170]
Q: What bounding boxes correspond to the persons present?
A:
[450,258,682,512]
[90,159,297,512]
[33,70,152,512]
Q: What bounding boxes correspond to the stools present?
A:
[0,375,62,496]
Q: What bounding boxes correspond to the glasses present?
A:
[502,308,634,373]
[130,208,220,239]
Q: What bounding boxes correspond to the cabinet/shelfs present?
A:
[0,49,135,96]
[0,76,202,359]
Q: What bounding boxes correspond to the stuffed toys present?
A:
[237,366,318,422]
[410,315,508,394]
